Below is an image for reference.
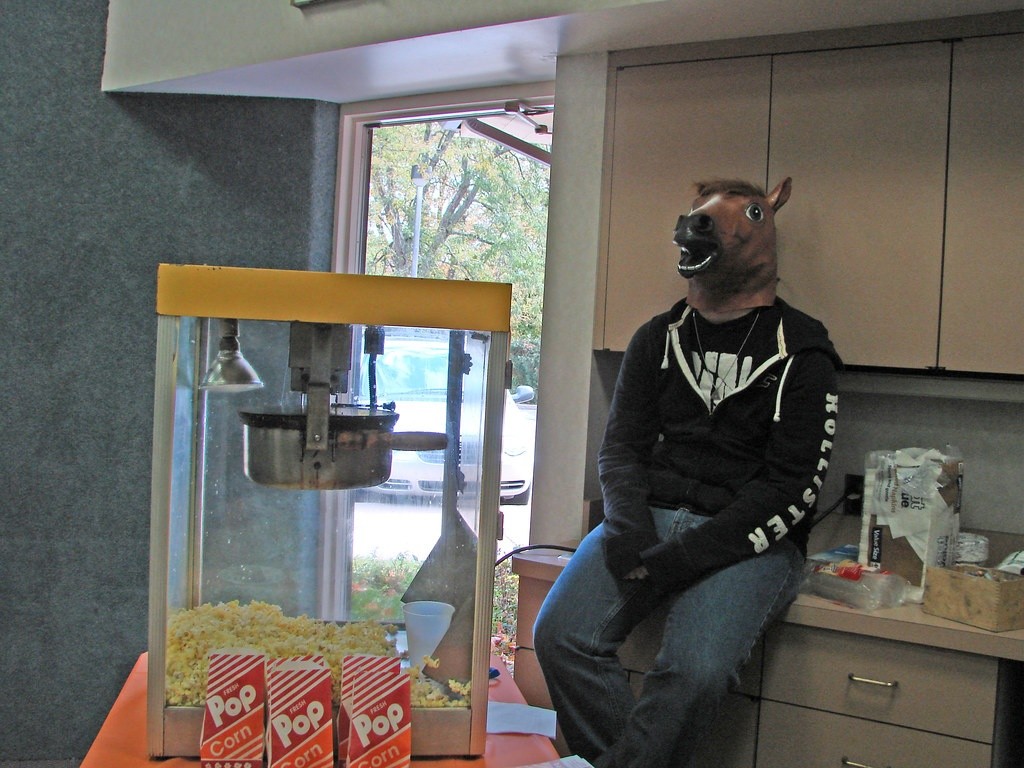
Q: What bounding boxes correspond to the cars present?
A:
[360,334,537,502]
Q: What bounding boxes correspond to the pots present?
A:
[240,393,398,493]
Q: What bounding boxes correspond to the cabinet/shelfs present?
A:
[510,536,764,768]
[751,619,1001,768]
[937,32,1024,376]
[604,52,773,354]
[764,38,954,371]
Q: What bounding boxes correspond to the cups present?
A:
[401,600,455,673]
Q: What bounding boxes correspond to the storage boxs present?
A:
[921,563,1024,632]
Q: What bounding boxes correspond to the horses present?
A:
[672,174,793,312]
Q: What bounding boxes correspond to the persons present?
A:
[534,192,843,766]
